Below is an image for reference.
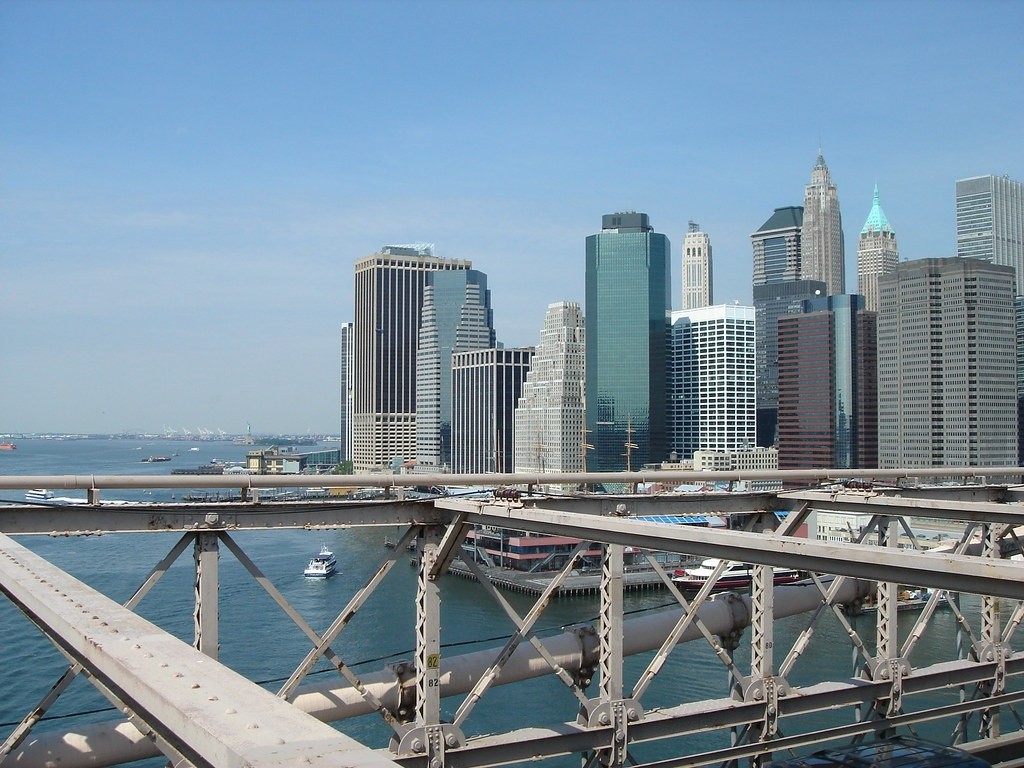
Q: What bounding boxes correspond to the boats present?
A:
[304,538,338,580]
[670,557,801,593]
[836,585,956,616]
[24,488,55,501]
